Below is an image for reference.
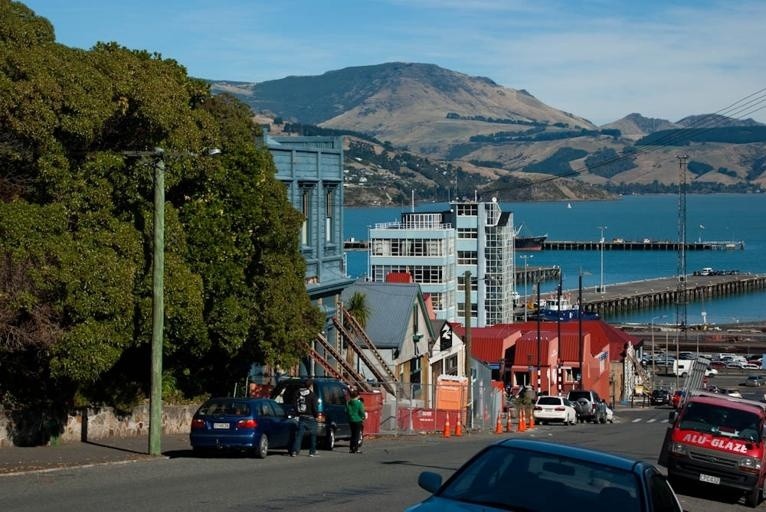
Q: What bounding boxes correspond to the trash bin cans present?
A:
[359,392,383,437]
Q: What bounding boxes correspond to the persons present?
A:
[290,378,321,458]
[344,390,368,454]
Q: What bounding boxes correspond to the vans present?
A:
[672,359,718,378]
[655,397,766,509]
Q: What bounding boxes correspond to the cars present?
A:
[190,395,302,458]
[650,390,672,407]
[534,300,546,307]
[403,436,687,512]
[718,387,744,400]
[642,350,766,371]
[533,396,577,427]
[604,403,613,423]
[700,268,712,276]
[744,375,765,387]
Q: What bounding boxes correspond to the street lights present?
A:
[146,143,222,456]
[651,314,668,393]
[519,254,533,323]
[597,223,607,293]
[675,326,690,393]
[462,269,492,430]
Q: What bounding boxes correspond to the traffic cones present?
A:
[495,410,502,433]
[522,408,527,430]
[506,410,512,432]
[516,408,524,432]
[442,410,450,438]
[455,410,463,437]
[528,409,534,428]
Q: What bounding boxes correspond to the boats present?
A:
[514,221,548,251]
[522,282,600,323]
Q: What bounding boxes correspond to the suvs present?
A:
[269,375,364,452]
[565,390,608,425]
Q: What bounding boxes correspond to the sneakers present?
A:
[292,452,298,457]
[308,450,320,458]
